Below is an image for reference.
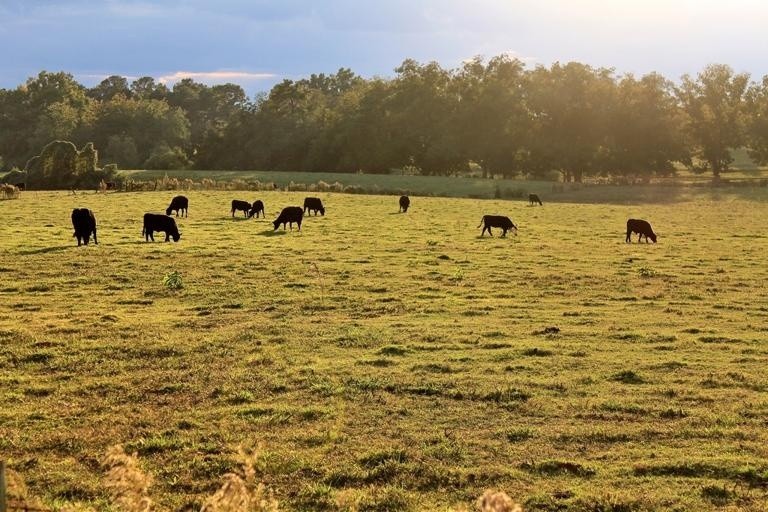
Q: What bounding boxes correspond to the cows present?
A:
[528,193,543,206]
[626,219,656,244]
[272,207,303,231]
[477,215,518,238]
[72,208,98,246]
[399,195,410,213]
[303,197,325,216]
[166,196,188,218]
[142,213,182,243]
[229,198,266,220]
